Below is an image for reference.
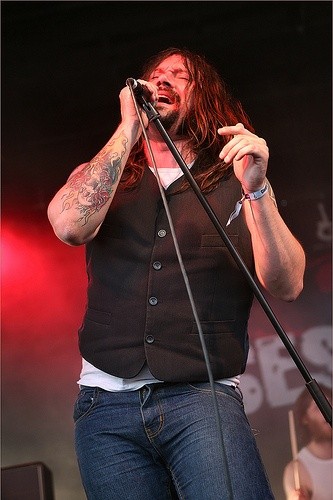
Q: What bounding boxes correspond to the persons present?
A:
[284,384,333,500]
[46,49,308,500]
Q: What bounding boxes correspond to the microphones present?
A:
[127,78,157,108]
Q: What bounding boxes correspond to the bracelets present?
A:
[226,182,270,229]
